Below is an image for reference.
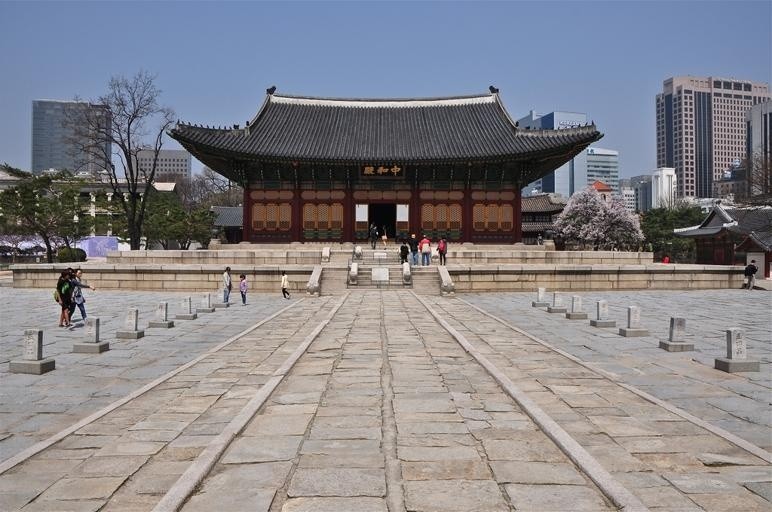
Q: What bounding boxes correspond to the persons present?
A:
[662,255,670,264]
[419,235,431,266]
[371,225,378,250]
[281,270,291,300]
[69,268,97,323]
[408,234,419,265]
[222,266,232,303]
[57,268,75,328]
[239,273,248,306]
[381,224,388,250]
[744,260,759,291]
[399,241,409,265]
[437,235,448,267]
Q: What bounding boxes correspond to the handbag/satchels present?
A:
[439,240,445,251]
[54,291,58,302]
[381,236,387,240]
